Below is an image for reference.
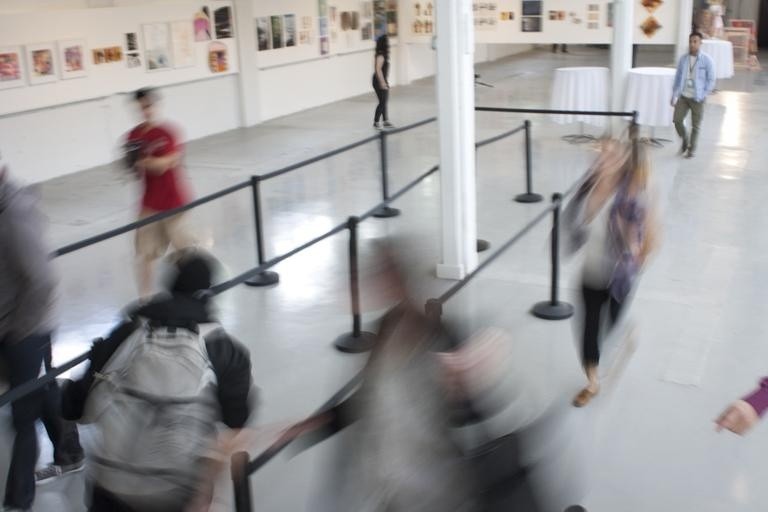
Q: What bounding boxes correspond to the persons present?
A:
[115,86,206,299]
[671,30,718,161]
[261,233,587,512]
[368,33,395,132]
[49,246,254,512]
[547,125,660,413]
[707,372,767,441]
[0,164,89,512]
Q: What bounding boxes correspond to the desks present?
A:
[699,39,735,94]
[550,66,609,144]
[621,66,677,148]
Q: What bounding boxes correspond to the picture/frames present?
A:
[0,37,88,90]
